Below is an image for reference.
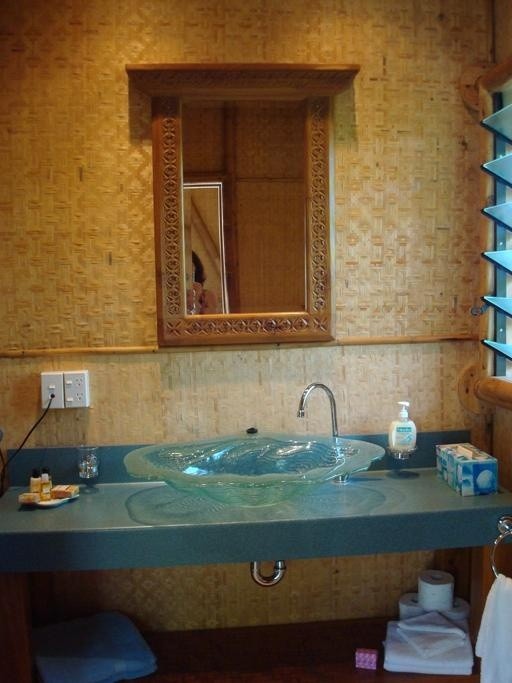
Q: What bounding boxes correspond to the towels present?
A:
[30,608,159,683]
[474,574,512,683]
[396,610,467,659]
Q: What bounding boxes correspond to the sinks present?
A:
[123,432,385,508]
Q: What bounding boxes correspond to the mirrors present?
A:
[126,61,360,349]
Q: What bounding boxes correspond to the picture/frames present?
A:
[183,182,232,315]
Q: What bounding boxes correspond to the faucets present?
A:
[297,381,352,436]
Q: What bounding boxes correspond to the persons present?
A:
[189,249,219,313]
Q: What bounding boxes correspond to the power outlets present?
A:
[61,372,91,409]
[41,372,64,410]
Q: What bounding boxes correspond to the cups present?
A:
[78,444,99,477]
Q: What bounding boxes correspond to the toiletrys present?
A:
[30,466,53,502]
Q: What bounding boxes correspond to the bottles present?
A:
[30,464,52,499]
